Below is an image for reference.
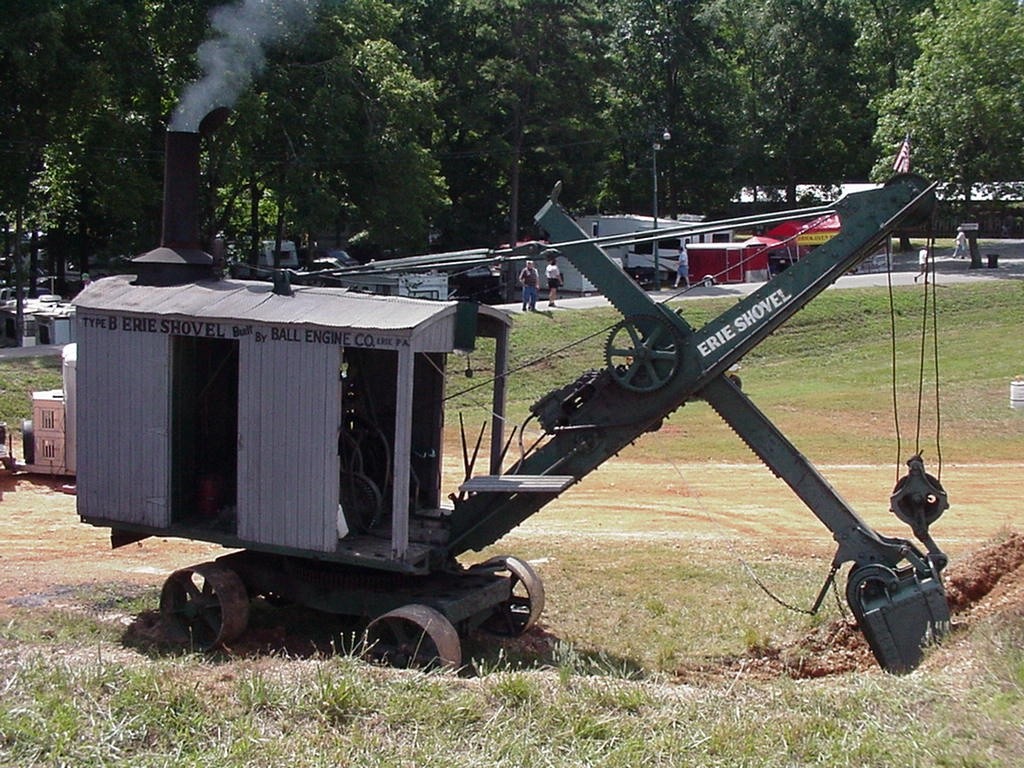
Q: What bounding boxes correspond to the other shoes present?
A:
[529,306,535,311]
[523,306,527,311]
[548,303,554,306]
[915,277,917,282]
[672,285,676,289]
[927,282,930,284]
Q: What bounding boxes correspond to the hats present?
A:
[957,227,962,231]
[921,246,927,250]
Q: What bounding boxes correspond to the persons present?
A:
[912,245,930,284]
[80,272,94,288]
[516,260,540,311]
[671,246,690,288]
[952,225,967,259]
[543,259,564,306]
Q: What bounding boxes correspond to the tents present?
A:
[745,214,842,279]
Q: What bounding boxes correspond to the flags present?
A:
[892,131,910,174]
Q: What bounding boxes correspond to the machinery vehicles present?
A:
[69,104,952,672]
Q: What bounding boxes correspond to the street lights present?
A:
[654,126,672,289]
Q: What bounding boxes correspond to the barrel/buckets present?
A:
[1010,381,1024,408]
[337,503,349,540]
[195,471,223,514]
